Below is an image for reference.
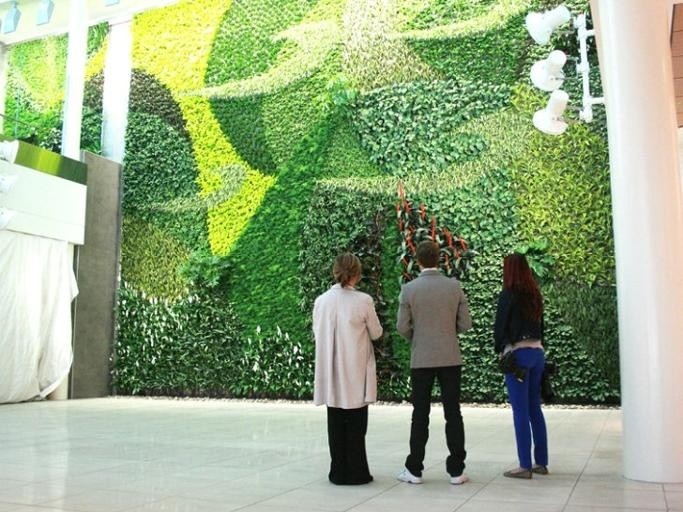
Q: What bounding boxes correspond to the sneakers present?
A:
[398,467,422,484]
[450,473,468,485]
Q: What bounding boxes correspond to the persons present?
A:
[492,252,550,479]
[311,251,383,485]
[395,241,474,485]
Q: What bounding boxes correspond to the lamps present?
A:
[2,1,53,34]
[524,0,604,138]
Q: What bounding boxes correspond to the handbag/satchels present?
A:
[499,351,527,383]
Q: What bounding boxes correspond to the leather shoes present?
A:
[504,466,548,479]
